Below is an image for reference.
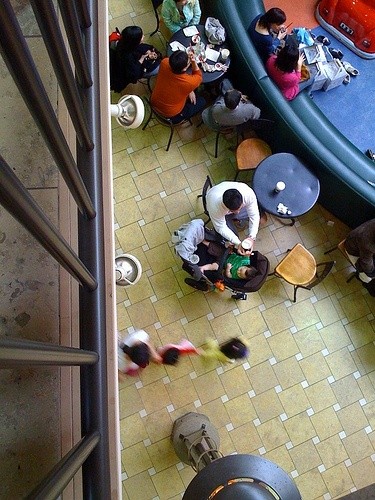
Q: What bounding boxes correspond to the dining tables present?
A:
[167,26,231,83]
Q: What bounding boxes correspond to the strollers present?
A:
[179,220,270,300]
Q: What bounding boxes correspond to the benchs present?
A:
[218,0,375,229]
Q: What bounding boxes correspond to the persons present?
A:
[158,339,197,365]
[266,45,315,100]
[211,79,260,125]
[206,181,260,255]
[345,218,375,284]
[150,50,202,123]
[199,240,257,280]
[247,8,288,62]
[110,26,157,92]
[118,330,162,383]
[161,0,202,32]
[201,337,251,364]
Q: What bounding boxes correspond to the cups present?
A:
[242,239,252,250]
[222,49,230,59]
[276,181,285,193]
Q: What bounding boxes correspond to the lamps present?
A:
[115,254,143,288]
[111,94,146,130]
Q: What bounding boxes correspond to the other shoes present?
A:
[329,48,343,59]
[317,36,331,45]
[342,61,359,83]
[184,278,208,291]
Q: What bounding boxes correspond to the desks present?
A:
[251,153,321,225]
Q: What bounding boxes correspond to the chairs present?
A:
[268,243,337,302]
[233,119,274,181]
[150,0,164,38]
[197,176,215,226]
[324,236,360,283]
[143,96,193,151]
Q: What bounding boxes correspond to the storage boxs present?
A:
[300,44,347,92]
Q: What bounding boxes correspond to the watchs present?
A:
[250,235,256,241]
